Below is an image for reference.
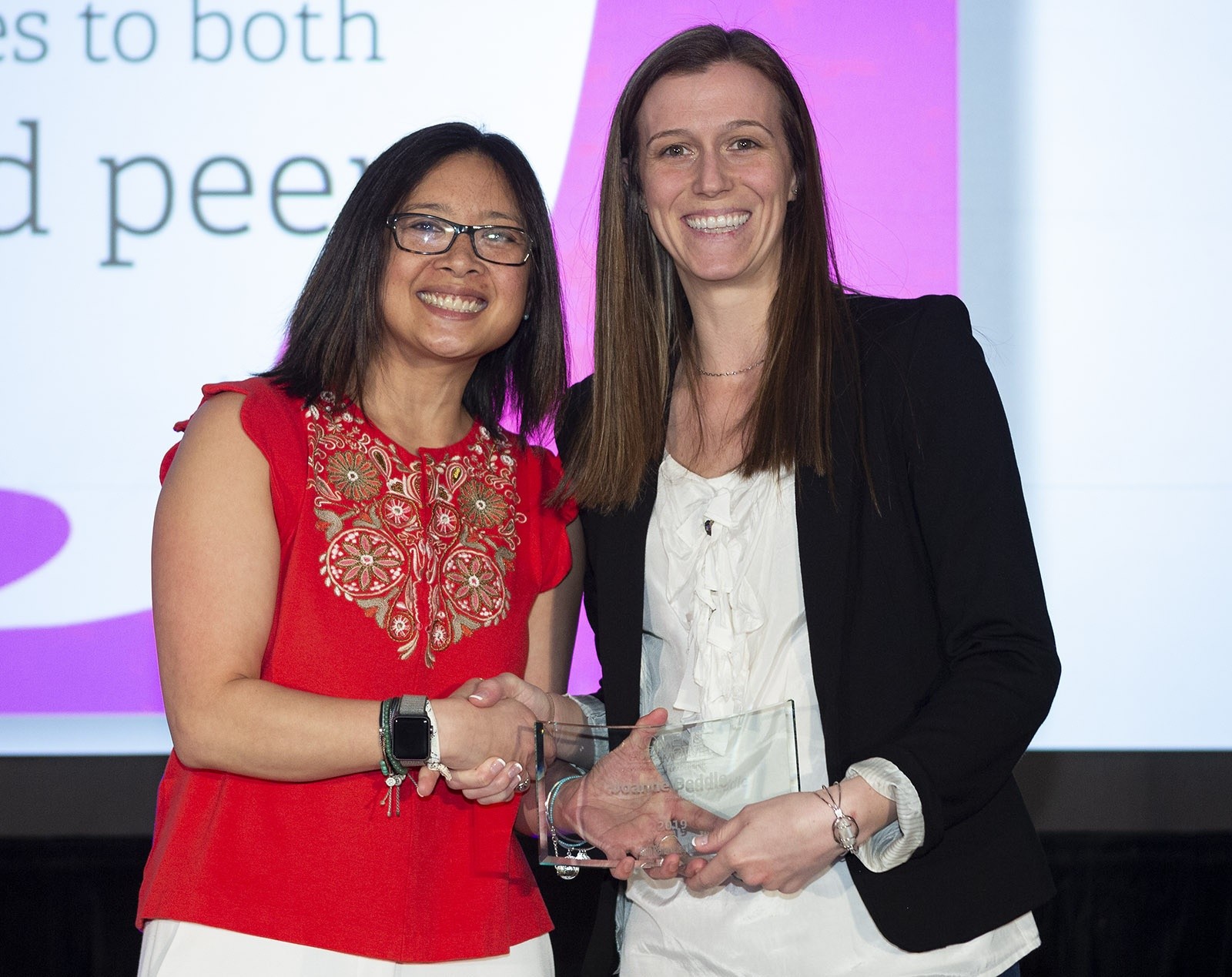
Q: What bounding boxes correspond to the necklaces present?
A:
[682,335,769,377]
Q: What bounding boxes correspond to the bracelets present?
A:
[563,692,609,762]
[378,693,453,817]
[543,774,596,880]
[811,781,860,859]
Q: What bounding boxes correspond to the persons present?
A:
[129,122,583,977]
[553,23,1067,977]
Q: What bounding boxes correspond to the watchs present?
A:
[392,694,434,767]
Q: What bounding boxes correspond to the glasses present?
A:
[385,212,536,266]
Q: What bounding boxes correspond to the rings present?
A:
[515,771,531,793]
[658,834,679,852]
[637,846,657,860]
